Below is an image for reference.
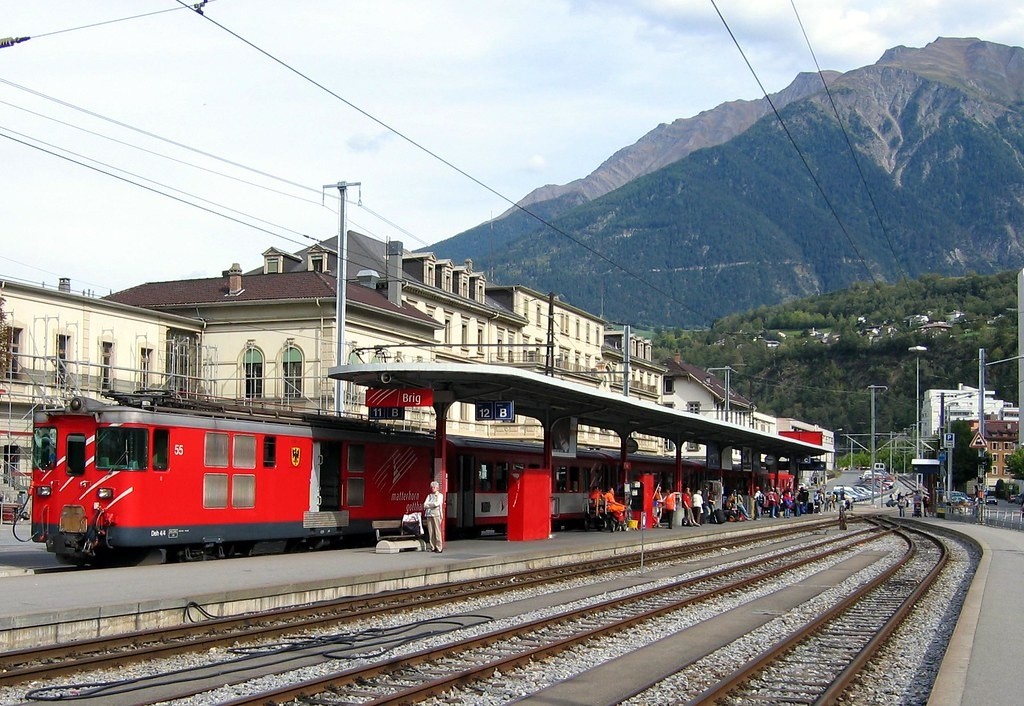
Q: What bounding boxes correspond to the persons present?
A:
[887,490,932,518]
[587,482,850,532]
[422,480,444,553]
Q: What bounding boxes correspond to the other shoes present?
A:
[818,512,822,516]
[689,522,699,527]
[773,515,776,518]
[431,547,443,552]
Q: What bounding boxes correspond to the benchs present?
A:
[372,520,416,541]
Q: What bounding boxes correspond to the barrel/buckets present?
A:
[627,520,638,528]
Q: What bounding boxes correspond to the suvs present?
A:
[798,483,808,491]
[864,470,872,474]
[834,487,866,501]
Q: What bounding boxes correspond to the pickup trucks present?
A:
[883,479,894,489]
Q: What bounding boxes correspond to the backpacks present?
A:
[768,492,775,502]
[757,493,766,506]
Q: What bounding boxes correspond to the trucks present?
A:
[874,463,885,472]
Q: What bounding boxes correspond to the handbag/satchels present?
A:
[814,494,819,501]
[402,517,419,536]
[739,512,745,522]
[727,511,738,522]
[785,499,793,507]
[682,516,690,525]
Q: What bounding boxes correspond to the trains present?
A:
[13,390,795,566]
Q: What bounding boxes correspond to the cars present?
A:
[852,488,866,501]
[985,496,998,505]
[857,487,873,498]
[855,479,889,492]
[1010,495,1016,503]
[826,491,858,504]
[822,490,853,503]
[936,490,973,506]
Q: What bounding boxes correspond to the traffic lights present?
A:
[814,476,818,485]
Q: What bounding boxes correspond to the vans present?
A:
[862,473,885,482]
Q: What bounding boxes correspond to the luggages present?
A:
[713,509,724,524]
